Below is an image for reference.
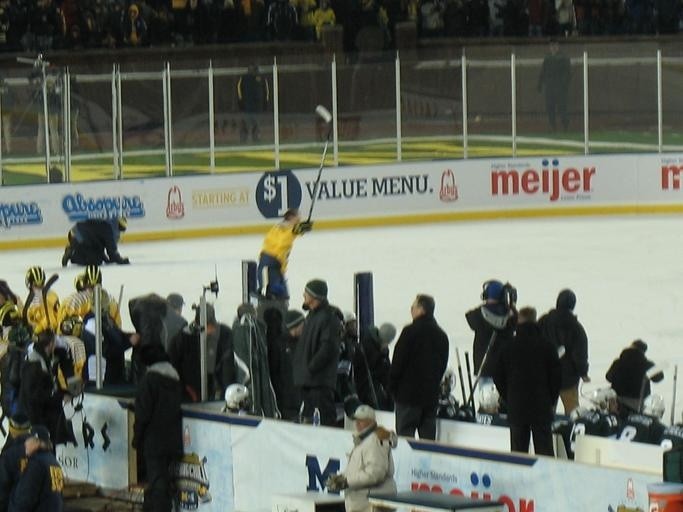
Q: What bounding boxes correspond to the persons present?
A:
[167,278,470,443]
[1,262,186,511]
[537,41,571,134]
[58,215,128,269]
[464,279,591,462]
[0,2,683,66]
[580,339,682,482]
[237,62,269,146]
[0,68,82,155]
[324,406,398,512]
[253,209,314,299]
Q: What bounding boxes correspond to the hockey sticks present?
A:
[307,105,333,223]
[22,273,59,338]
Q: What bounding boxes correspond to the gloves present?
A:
[293,221,313,235]
[327,474,349,489]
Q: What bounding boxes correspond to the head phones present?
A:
[71,391,84,412]
[482,280,490,300]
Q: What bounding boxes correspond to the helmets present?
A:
[83,264,102,287]
[25,265,45,288]
[225,383,249,410]
[58,314,84,336]
[480,280,506,301]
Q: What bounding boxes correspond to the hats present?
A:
[305,280,327,300]
[28,425,51,441]
[119,217,127,230]
[354,404,376,420]
[286,310,304,328]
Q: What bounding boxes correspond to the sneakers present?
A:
[62,246,71,266]
